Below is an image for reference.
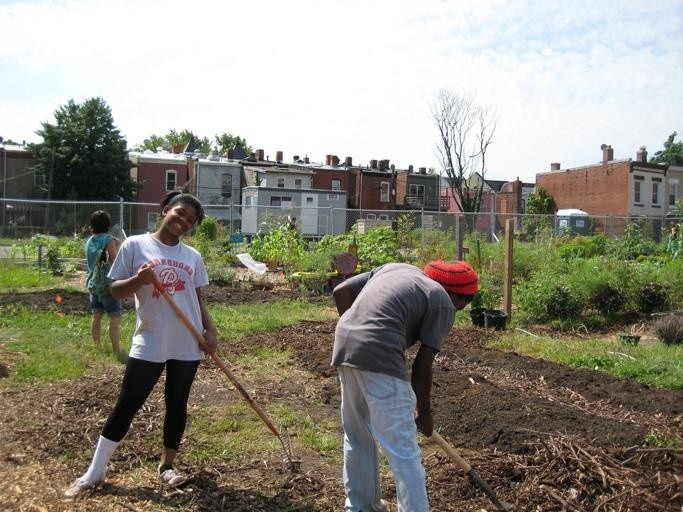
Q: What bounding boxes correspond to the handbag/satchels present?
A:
[91,251,114,296]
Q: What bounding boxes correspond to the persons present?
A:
[327,253,481,511]
[84,207,129,360]
[65,189,219,500]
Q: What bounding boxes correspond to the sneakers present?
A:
[156,466,186,486]
[65,478,101,497]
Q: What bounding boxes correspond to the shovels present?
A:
[413,410,515,512]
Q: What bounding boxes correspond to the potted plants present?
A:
[619,321,645,347]
[470,284,508,330]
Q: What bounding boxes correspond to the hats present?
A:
[424,260,478,295]
